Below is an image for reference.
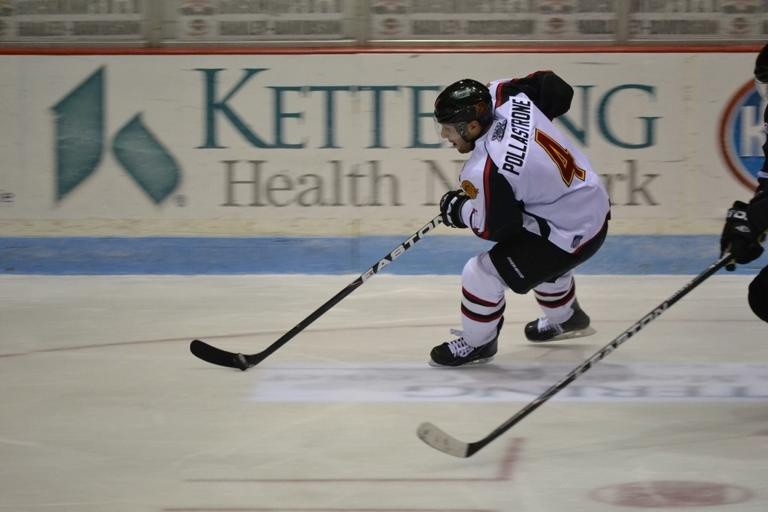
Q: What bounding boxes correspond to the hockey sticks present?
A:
[190,213,444,371]
[417,253,734,460]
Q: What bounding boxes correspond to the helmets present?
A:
[433,78,493,152]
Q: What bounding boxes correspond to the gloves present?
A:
[720,191,768,271]
[437,188,471,232]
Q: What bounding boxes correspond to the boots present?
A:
[524,296,590,341]
[428,316,505,367]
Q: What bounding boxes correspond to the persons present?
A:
[431,69,610,368]
[717,44,767,324]
